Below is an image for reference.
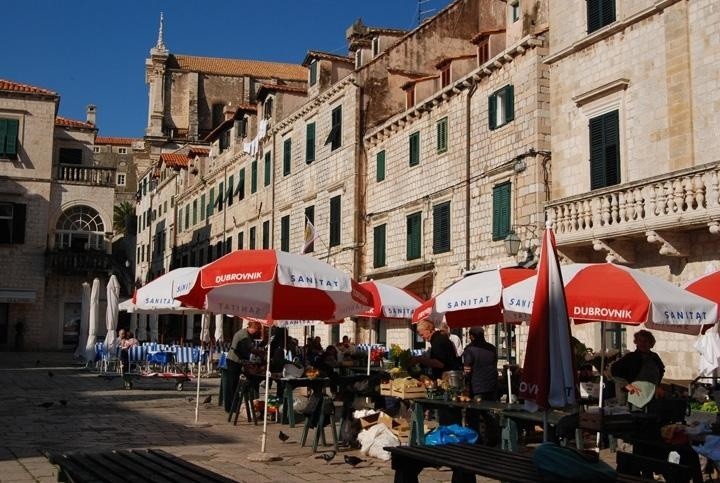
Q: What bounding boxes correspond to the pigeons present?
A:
[315,450,336,465]
[203,395,212,404]
[279,431,289,442]
[38,402,54,411]
[48,369,56,378]
[344,455,367,468]
[60,399,69,406]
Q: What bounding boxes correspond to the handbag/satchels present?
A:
[426,424,479,445]
[234,350,250,360]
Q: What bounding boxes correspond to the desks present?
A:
[503,407,579,453]
[219,367,333,430]
[413,399,505,449]
[334,365,408,412]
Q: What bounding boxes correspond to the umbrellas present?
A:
[411,264,539,404]
[685,270,720,336]
[518,220,580,443]
[503,256,719,453]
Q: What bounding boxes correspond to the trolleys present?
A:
[120,349,192,392]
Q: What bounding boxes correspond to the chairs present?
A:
[94,342,210,378]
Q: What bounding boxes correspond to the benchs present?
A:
[44,448,243,483]
[383,442,665,483]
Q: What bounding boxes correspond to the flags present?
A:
[302,220,321,254]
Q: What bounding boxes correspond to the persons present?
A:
[409,320,462,426]
[438,323,464,362]
[464,327,498,400]
[610,330,665,413]
[226,321,356,413]
